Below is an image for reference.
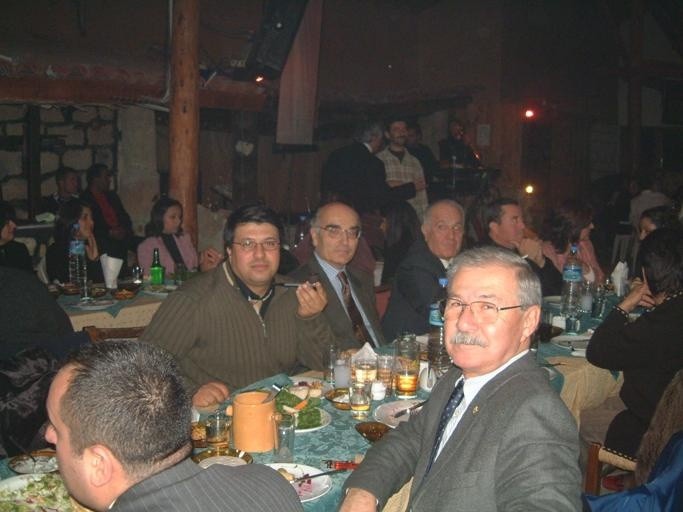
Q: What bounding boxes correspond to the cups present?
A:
[271,411,296,466]
[373,261,387,288]
[174,262,188,285]
[319,347,397,420]
[128,262,145,284]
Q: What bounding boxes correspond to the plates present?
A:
[356,399,424,441]
[263,462,334,504]
[285,376,334,434]
[57,284,177,300]
[7,451,60,476]
[551,333,594,351]
[191,448,254,470]
[544,293,563,310]
[1,473,55,505]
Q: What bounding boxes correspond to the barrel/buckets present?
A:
[224,391,283,454]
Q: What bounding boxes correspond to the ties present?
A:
[337,271,376,350]
[423,380,464,482]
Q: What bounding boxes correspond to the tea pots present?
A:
[230,390,280,454]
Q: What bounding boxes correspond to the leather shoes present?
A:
[602,475,623,492]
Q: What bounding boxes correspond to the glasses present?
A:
[319,225,361,239]
[439,299,522,325]
[231,240,282,250]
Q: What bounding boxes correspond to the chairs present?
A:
[1,322,147,458]
[580,362,681,512]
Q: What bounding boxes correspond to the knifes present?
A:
[391,398,429,418]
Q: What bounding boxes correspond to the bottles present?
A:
[559,243,584,319]
[191,407,230,450]
[391,329,420,397]
[577,278,607,318]
[150,247,163,285]
[68,222,88,291]
[427,278,450,374]
